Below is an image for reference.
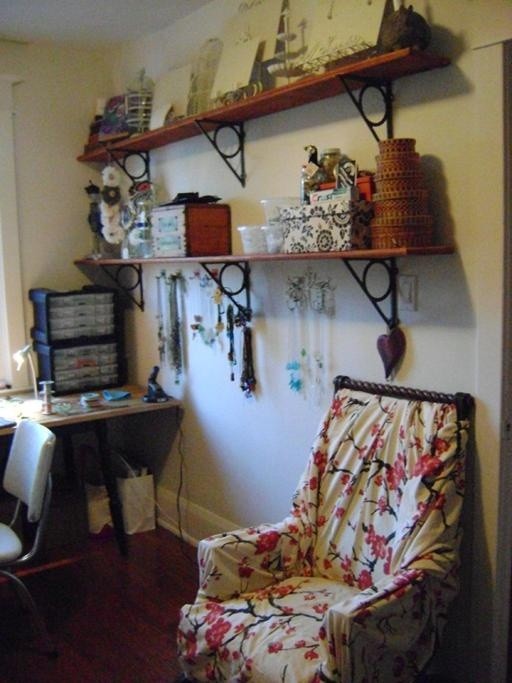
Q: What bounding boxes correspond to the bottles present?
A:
[320,146,342,183]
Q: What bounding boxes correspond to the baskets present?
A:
[369,138,434,250]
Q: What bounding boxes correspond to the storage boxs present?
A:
[279,199,372,254]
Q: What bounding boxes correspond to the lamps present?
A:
[13,344,39,399]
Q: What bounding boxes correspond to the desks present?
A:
[0,382,183,557]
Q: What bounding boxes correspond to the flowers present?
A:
[99,166,123,245]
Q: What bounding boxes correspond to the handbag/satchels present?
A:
[111,474,156,536]
[85,483,116,542]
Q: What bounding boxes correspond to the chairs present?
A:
[175,373,476,681]
[0,418,57,658]
